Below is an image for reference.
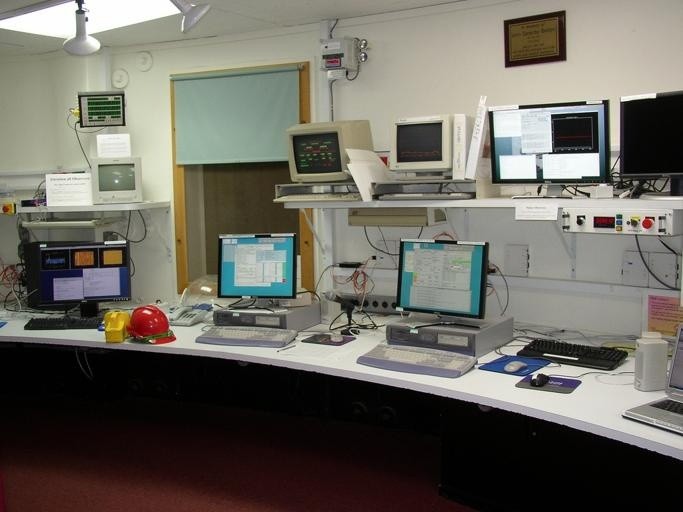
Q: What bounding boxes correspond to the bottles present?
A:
[634,331,669,392]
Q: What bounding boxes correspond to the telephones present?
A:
[169,307,208,326]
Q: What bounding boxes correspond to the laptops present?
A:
[622,325,683,436]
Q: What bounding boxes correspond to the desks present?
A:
[0,303,682,464]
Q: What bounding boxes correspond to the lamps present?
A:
[168,1,210,33]
[62,1,101,58]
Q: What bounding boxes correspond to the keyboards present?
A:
[516,339,629,371]
[195,326,297,348]
[357,343,477,379]
[24,317,102,330]
[274,192,359,201]
[379,191,475,200]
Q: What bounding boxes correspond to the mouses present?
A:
[530,372,549,386]
[330,332,343,343]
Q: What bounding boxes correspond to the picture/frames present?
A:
[503,10,568,68]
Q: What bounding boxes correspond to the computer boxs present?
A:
[214,301,324,331]
[386,314,515,357]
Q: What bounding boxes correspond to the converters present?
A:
[340,262,361,268]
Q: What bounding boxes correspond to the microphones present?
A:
[324,291,343,304]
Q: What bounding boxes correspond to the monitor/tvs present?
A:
[286,119,372,183]
[37,240,132,317]
[488,98,612,200]
[390,115,453,179]
[396,238,490,329]
[77,90,127,128]
[619,89,683,199]
[217,232,298,313]
[89,156,143,204]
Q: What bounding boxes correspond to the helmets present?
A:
[126,305,175,345]
